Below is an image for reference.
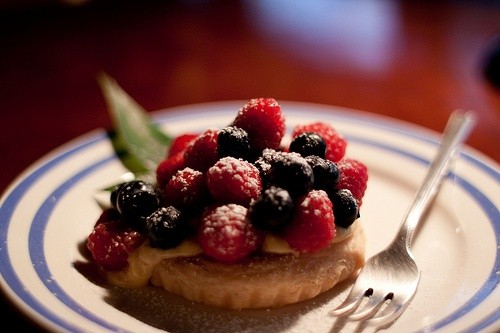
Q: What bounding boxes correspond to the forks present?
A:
[329,109,477,326]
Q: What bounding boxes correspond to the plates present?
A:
[1,100,500,333]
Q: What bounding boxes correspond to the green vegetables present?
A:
[95,74,170,192]
